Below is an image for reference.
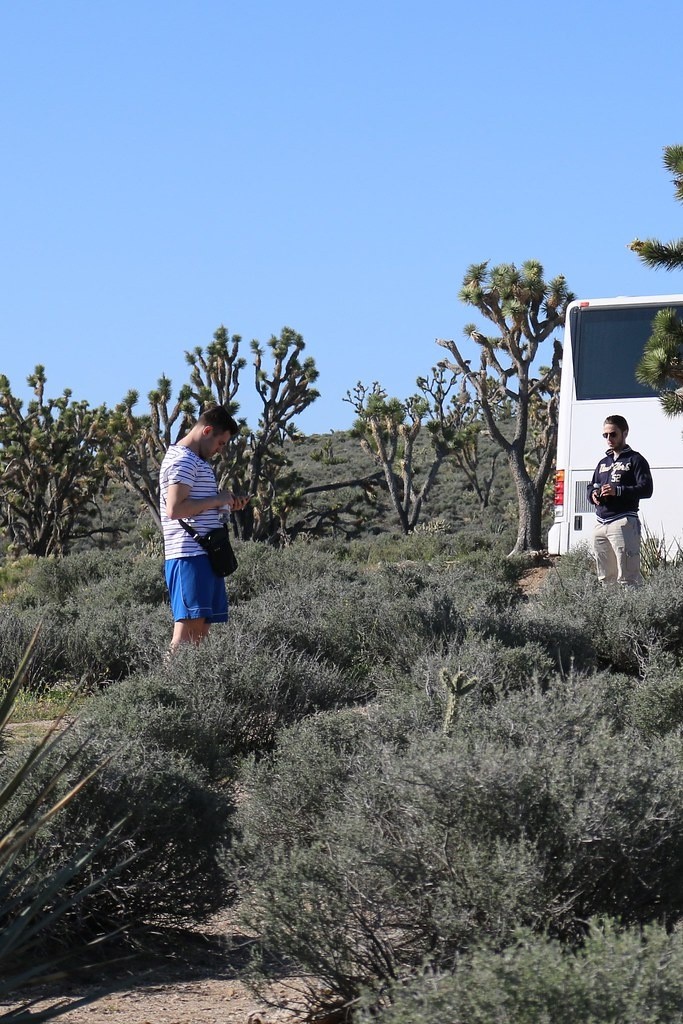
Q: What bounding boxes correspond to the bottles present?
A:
[218,504,231,523]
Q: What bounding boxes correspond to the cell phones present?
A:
[240,494,254,502]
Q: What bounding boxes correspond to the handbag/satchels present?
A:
[203,527,238,578]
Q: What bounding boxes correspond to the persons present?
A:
[586,415,653,587]
[159,406,251,663]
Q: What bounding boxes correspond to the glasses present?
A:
[603,430,625,438]
